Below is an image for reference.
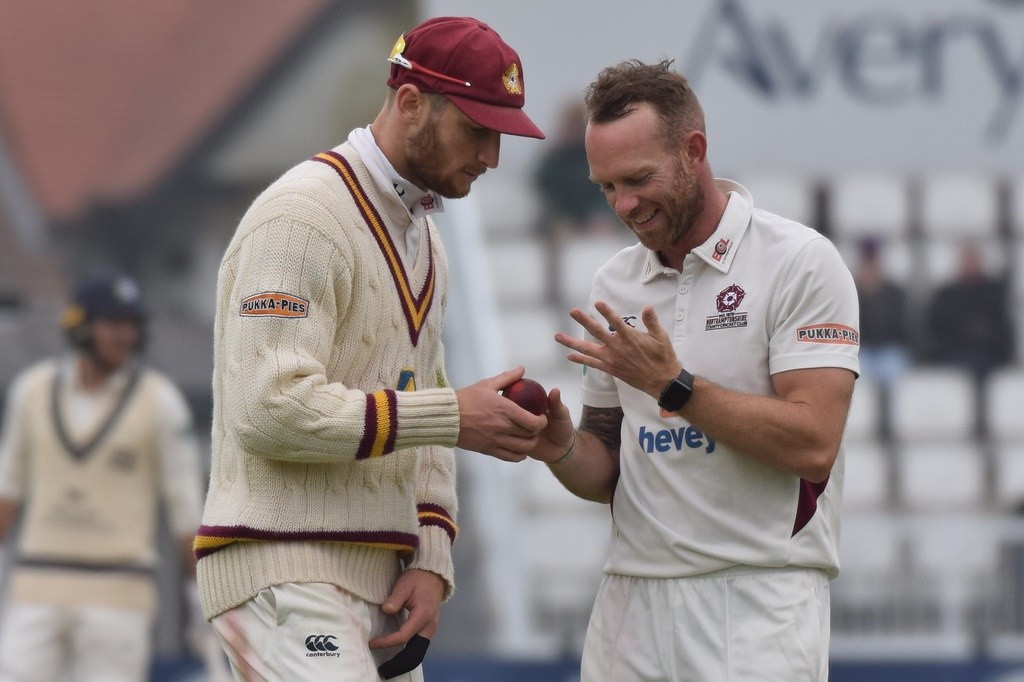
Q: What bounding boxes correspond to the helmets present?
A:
[61,271,152,354]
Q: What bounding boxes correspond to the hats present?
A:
[385,14,546,141]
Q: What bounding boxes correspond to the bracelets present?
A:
[545,428,576,465]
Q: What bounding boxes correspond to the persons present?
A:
[846,232,1015,445]
[531,59,860,682]
[0,276,204,681]
[193,14,545,682]
[530,97,628,316]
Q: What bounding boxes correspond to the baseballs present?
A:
[501,379,550,416]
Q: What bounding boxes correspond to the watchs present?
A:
[658,369,694,413]
